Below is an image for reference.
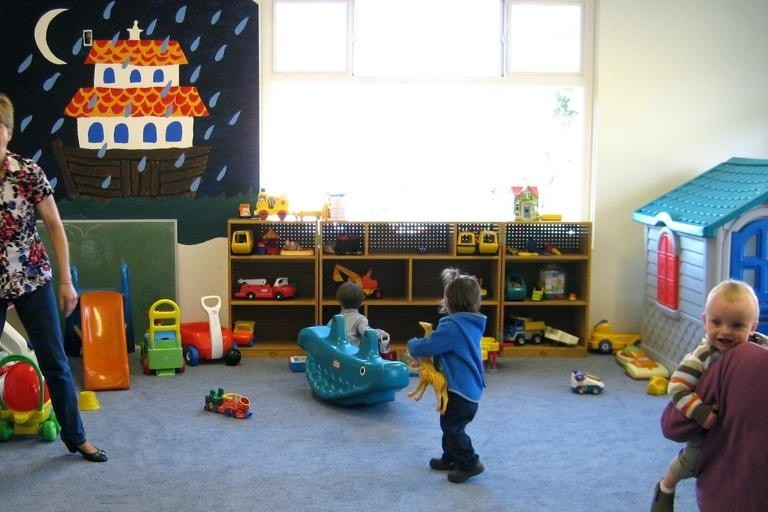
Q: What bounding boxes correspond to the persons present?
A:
[407,267,488,484]
[326,282,390,348]
[1,95,108,464]
[650,279,768,512]
[659,340,768,512]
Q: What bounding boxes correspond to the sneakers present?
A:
[429,458,455,470]
[448,460,484,483]
[650,478,674,512]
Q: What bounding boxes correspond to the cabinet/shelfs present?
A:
[499,221,594,357]
[319,221,502,362]
[227,219,317,357]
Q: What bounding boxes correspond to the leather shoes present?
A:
[75,441,108,462]
